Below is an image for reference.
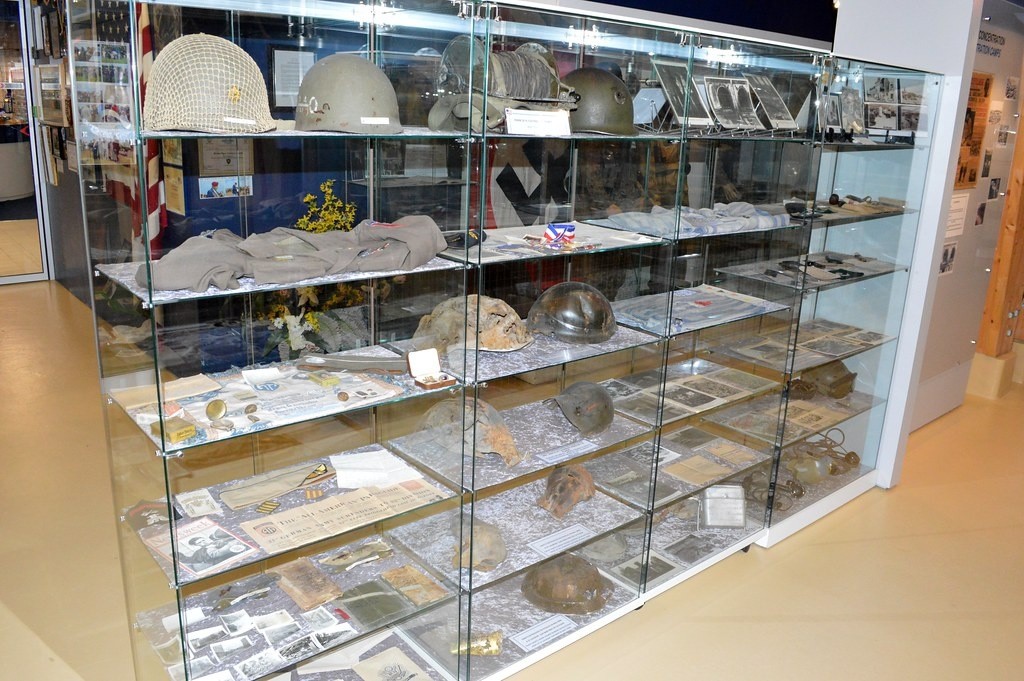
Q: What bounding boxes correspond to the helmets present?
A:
[144,32,277,135]
[559,67,640,137]
[526,280,618,345]
[800,359,857,388]
[543,380,615,436]
[294,53,404,134]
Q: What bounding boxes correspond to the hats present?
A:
[521,553,615,614]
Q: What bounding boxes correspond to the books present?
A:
[142,516,260,578]
[175,487,221,517]
[264,555,458,681]
[827,100,840,126]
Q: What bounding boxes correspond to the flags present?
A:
[132,0,169,350]
[470,138,572,228]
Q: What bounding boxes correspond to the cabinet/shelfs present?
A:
[20,0,946,681]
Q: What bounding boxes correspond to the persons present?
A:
[169,528,252,573]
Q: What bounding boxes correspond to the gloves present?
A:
[444,228,487,249]
